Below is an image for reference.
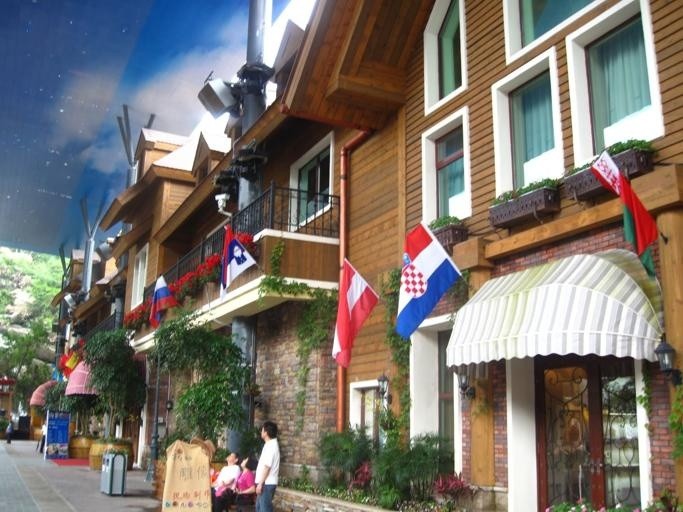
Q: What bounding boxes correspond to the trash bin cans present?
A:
[101,449,128,495]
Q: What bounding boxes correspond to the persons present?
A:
[214,454,258,511]
[209,451,241,511]
[39,419,48,454]
[3,421,14,444]
[253,421,281,511]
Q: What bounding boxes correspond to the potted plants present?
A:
[149,299,252,503]
[489,178,559,230]
[427,215,467,248]
[564,135,658,201]
[64,325,136,469]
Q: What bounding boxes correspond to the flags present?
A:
[617,173,657,279]
[393,224,459,339]
[149,274,177,328]
[217,226,254,301]
[329,256,378,368]
